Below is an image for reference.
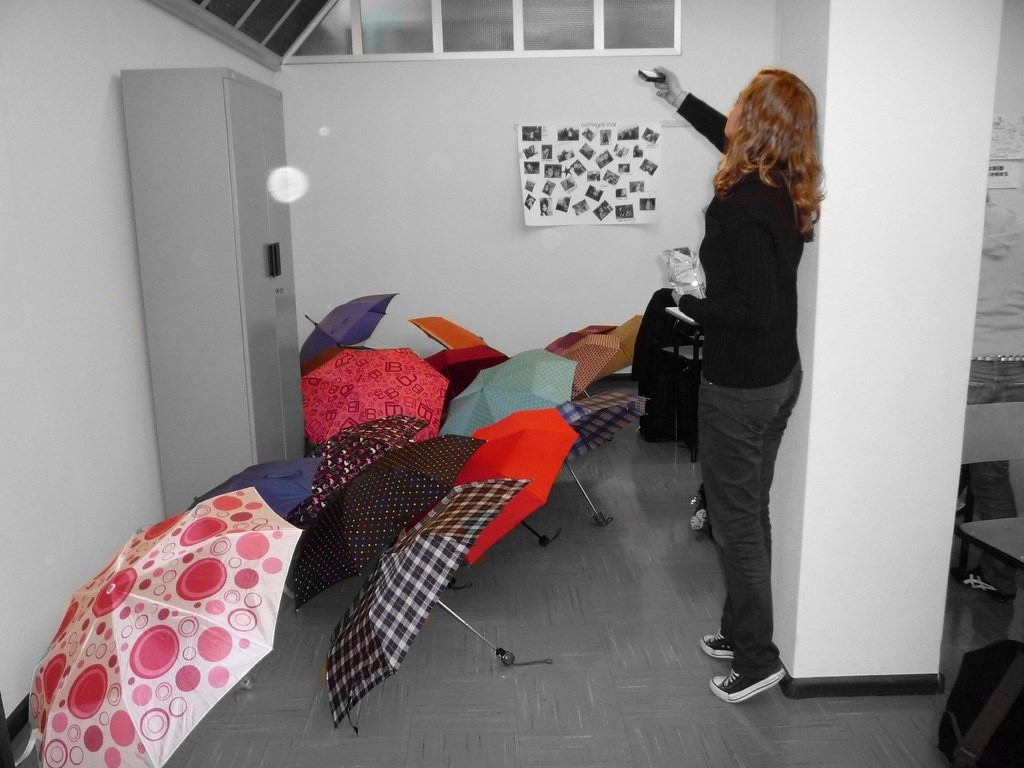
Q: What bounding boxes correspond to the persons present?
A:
[946,195,1023,601]
[649,68,822,702]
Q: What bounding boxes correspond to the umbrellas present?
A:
[186,456,329,519]
[545,315,645,441]
[326,479,553,737]
[294,434,486,611]
[288,416,431,526]
[454,409,579,565]
[30,486,305,768]
[302,346,450,442]
[439,350,578,438]
[556,391,647,526]
[300,293,509,407]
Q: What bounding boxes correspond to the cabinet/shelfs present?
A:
[119,66,303,522]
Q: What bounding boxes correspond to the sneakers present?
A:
[700,627,735,660]
[709,663,785,703]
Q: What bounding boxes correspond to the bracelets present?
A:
[671,91,685,106]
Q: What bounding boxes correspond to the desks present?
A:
[956,515,1024,768]
[665,308,706,463]
